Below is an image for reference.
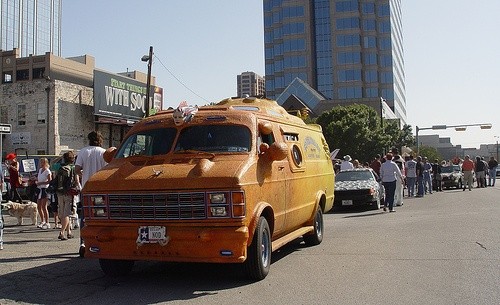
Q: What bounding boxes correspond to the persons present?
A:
[173,102,198,126]
[340,146,498,207]
[0,131,109,258]
[380,153,405,212]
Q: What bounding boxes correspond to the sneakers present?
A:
[43,223,50,229]
[37,222,45,227]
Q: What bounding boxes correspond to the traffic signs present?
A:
[0,124,12,135]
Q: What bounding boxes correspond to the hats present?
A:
[6,153,15,161]
[343,155,352,161]
[63,151,74,162]
[384,153,394,158]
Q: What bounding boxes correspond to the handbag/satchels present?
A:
[46,175,58,194]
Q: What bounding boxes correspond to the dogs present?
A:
[5,200,38,226]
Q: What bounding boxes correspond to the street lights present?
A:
[140,45,154,148]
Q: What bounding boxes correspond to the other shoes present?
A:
[383,202,403,212]
[67,234,74,238]
[58,232,68,240]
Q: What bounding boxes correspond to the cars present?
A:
[334,167,386,210]
[496,164,500,175]
[441,165,474,189]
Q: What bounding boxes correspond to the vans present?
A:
[76,94,336,282]
